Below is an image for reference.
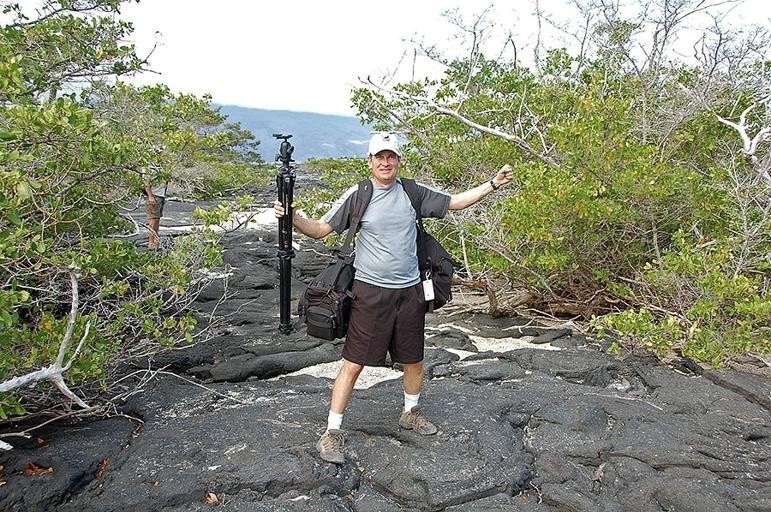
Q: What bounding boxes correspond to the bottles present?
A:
[369,134,401,157]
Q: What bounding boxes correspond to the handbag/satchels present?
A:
[490,179,500,193]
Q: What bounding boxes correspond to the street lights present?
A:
[298,263,355,341]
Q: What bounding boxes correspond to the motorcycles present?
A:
[271,133,296,325]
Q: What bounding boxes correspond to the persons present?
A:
[141,147,169,252]
[273,132,517,467]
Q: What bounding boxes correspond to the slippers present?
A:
[401,178,462,313]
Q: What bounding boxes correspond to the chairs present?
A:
[316,429,348,463]
[399,405,437,435]
